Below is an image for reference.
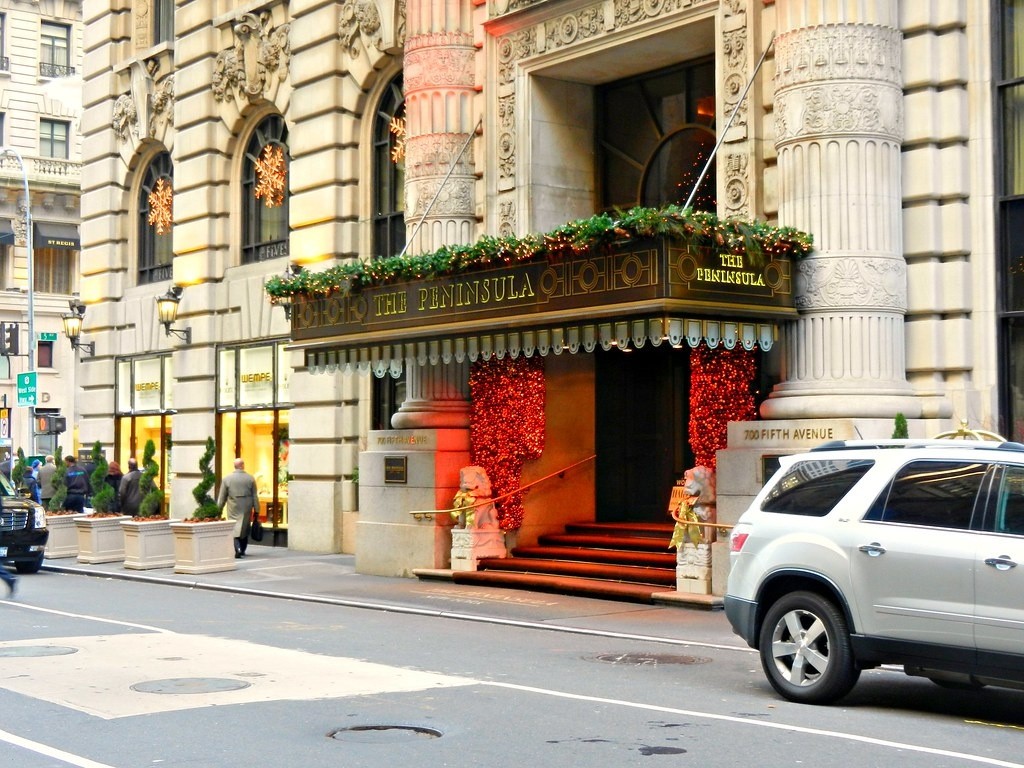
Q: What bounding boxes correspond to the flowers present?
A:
[264,202,815,298]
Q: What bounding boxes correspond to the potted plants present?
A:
[72,440,132,565]
[169,436,239,575]
[40,447,88,559]
[118,440,183,570]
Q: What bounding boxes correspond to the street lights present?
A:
[0,149,36,457]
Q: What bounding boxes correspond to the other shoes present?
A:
[235,548,242,559]
[240,551,245,556]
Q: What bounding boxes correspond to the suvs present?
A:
[722,436,1024,703]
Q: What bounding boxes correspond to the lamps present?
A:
[276,263,305,322]
[61,300,95,357]
[154,285,191,344]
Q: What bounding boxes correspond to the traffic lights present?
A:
[6,324,19,354]
[33,413,49,436]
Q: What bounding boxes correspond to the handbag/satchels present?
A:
[252,516,264,542]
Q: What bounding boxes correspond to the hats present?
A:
[64,455,78,460]
[32,459,40,468]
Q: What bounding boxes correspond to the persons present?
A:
[23,455,150,516]
[217,458,261,558]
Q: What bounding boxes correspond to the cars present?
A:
[0,472,50,574]
